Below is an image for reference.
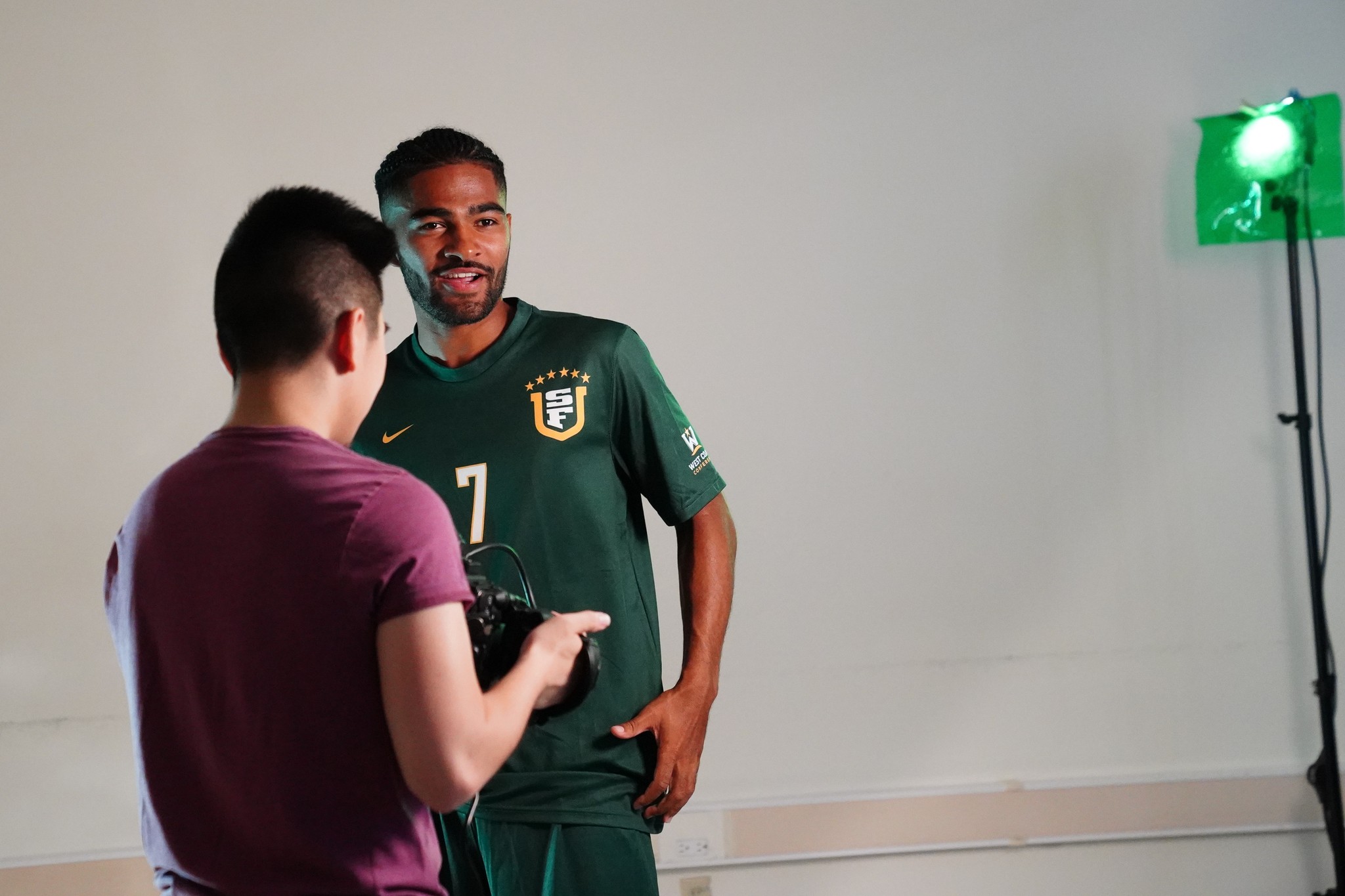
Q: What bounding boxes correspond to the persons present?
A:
[100,185,612,896]
[346,124,737,896]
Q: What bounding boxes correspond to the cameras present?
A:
[462,562,598,721]
